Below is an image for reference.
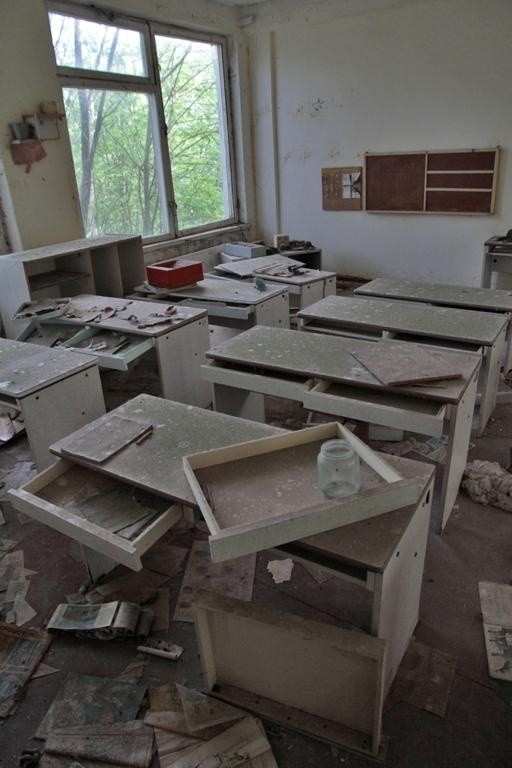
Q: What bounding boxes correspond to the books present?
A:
[61,412,153,465]
[478,579,512,681]
[349,342,465,387]
[0,599,281,767]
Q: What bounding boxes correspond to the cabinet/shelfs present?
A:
[1,231,145,342]
[360,147,501,218]
[220,240,322,272]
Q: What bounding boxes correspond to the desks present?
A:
[213,254,337,330]
[200,325,484,536]
[297,294,510,439]
[6,393,437,757]
[1,337,107,475]
[127,277,290,348]
[481,236,511,289]
[353,277,511,377]
[15,294,218,412]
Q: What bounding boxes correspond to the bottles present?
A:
[316,440,361,500]
